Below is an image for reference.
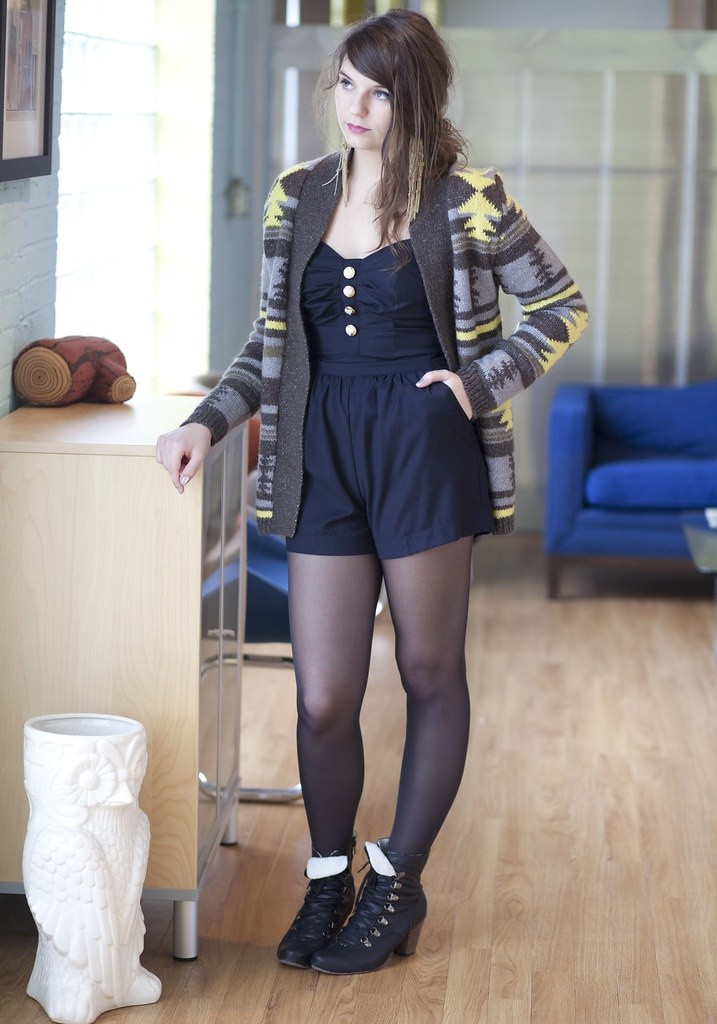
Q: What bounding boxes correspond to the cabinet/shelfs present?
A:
[0,396,247,961]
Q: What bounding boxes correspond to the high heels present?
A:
[276,830,357,968]
[311,837,432,974]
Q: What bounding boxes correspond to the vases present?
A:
[23,713,162,1024]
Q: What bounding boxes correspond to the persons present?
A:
[155,9,587,977]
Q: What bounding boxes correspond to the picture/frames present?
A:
[0,0,56,183]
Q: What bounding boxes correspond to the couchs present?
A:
[545,380,717,599]
